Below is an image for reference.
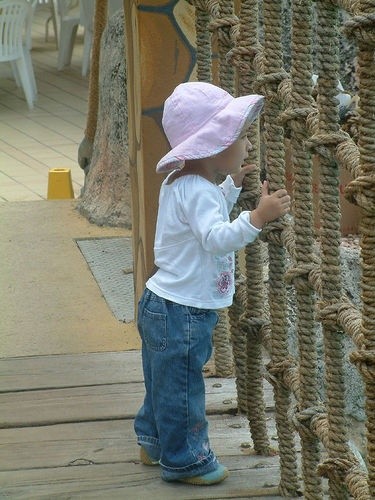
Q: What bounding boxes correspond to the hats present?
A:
[156,81,265,173]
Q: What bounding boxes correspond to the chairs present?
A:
[0,0,124,109]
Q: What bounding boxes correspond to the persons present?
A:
[127,81,291,487]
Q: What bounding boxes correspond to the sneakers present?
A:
[140,446,163,466]
[161,463,230,486]
